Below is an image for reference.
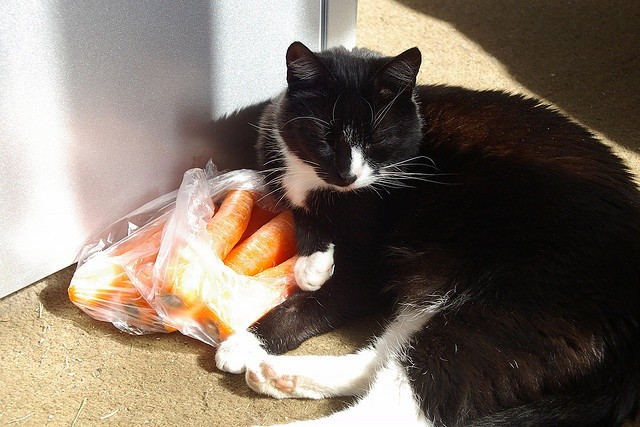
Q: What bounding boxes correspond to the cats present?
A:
[214,40,640,427]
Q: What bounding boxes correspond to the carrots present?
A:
[165,187,248,311]
[217,210,299,273]
